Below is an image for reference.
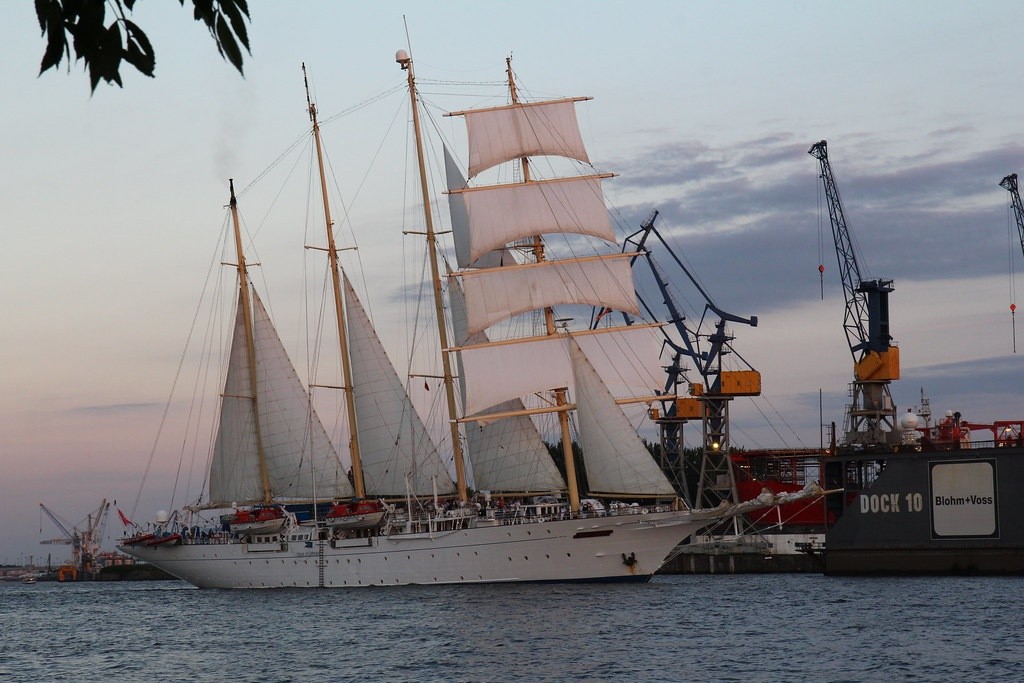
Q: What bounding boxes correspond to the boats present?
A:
[229,506,286,535]
[325,501,385,530]
[22,577,38,584]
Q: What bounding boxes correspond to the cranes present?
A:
[809,139,900,450]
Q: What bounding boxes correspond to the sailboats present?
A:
[114,15,850,592]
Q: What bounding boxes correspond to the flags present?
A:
[117,507,132,529]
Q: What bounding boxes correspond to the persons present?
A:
[186,529,214,539]
[414,496,517,517]
